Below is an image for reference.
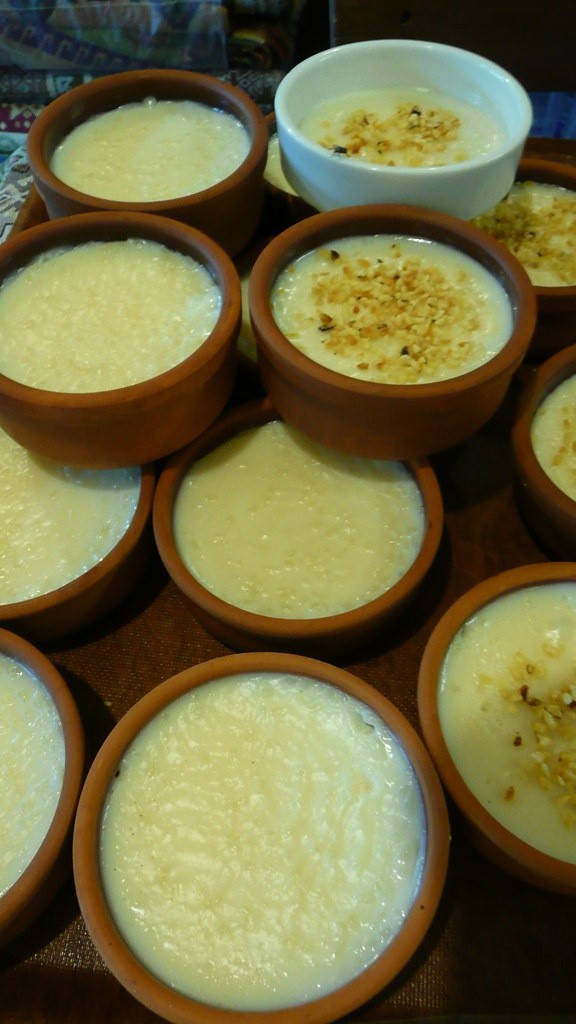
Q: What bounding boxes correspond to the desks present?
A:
[0,134,576,1024]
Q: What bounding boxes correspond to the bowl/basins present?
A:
[0,39,576,1024]
